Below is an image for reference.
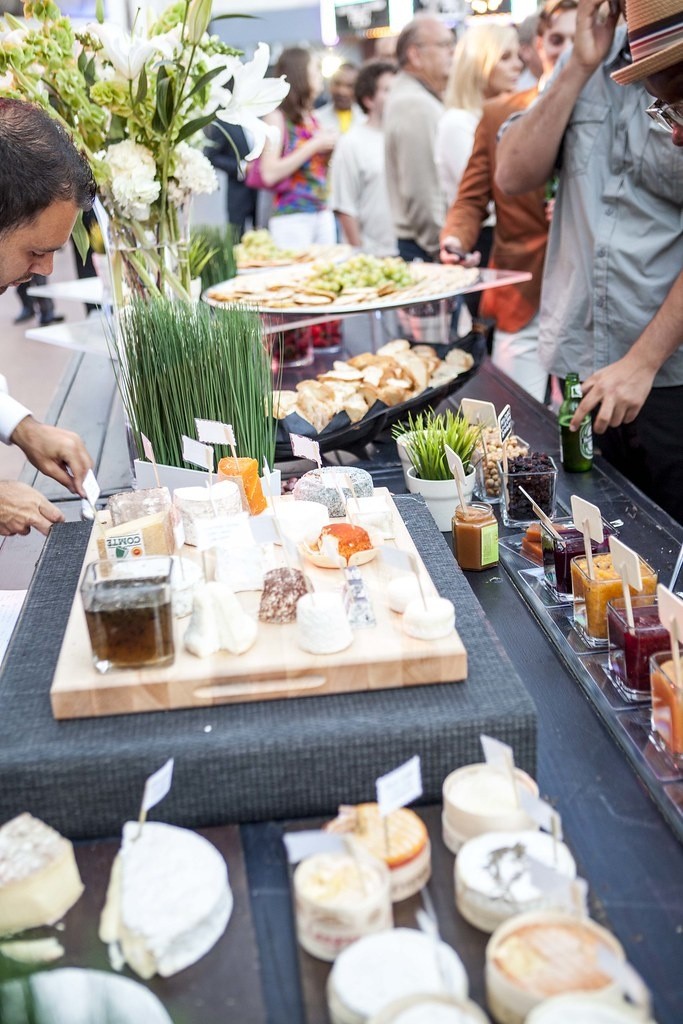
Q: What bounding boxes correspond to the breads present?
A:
[261,337,474,433]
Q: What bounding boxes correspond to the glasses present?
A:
[645,92,683,133]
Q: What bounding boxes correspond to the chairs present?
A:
[267,208,338,254]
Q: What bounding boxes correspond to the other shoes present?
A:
[16,308,35,322]
[41,314,65,326]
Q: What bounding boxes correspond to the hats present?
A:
[610,0,683,85]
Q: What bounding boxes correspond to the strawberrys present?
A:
[311,320,342,347]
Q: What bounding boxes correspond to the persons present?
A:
[0,98,98,537]
[496,0,683,534]
[309,63,358,245]
[383,17,454,264]
[330,64,408,259]
[260,48,341,250]
[432,21,524,320]
[439,0,583,405]
[202,74,259,244]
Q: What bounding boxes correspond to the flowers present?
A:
[0,0,293,226]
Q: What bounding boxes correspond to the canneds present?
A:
[452,502,499,571]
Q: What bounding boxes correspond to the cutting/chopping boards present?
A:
[49,486,471,720]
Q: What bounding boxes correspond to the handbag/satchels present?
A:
[246,108,292,191]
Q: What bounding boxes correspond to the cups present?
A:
[570,552,658,648]
[81,557,177,669]
[469,434,529,505]
[497,456,559,527]
[540,515,620,602]
[606,594,683,702]
[313,312,346,354]
[649,649,683,781]
[262,313,314,370]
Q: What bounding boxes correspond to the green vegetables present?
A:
[241,227,292,260]
[308,254,414,293]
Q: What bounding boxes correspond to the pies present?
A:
[237,252,312,266]
[206,284,399,305]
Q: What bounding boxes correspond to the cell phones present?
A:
[607,0,618,15]
[445,246,470,259]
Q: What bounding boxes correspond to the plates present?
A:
[21,239,533,461]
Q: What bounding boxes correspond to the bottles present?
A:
[559,373,594,473]
[398,293,472,344]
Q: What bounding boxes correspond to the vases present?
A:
[86,185,193,481]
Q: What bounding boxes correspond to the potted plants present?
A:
[390,406,497,534]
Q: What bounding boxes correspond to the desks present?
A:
[0,241,683,1024]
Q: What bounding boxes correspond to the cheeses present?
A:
[98,820,234,980]
[97,466,455,656]
[0,812,85,939]
